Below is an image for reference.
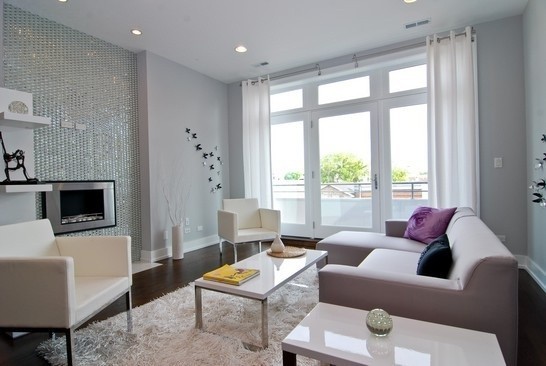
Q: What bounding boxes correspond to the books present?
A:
[203,264,260,286]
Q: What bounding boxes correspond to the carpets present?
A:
[39,263,321,365]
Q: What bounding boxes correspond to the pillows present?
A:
[417,234,452,277]
[404,207,458,242]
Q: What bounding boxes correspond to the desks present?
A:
[282,302,506,366]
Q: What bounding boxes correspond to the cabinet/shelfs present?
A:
[0,111,53,195]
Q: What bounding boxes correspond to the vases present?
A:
[172,226,184,258]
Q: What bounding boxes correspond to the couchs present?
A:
[316,208,519,366]
[217,199,282,259]
[0,217,133,366]
